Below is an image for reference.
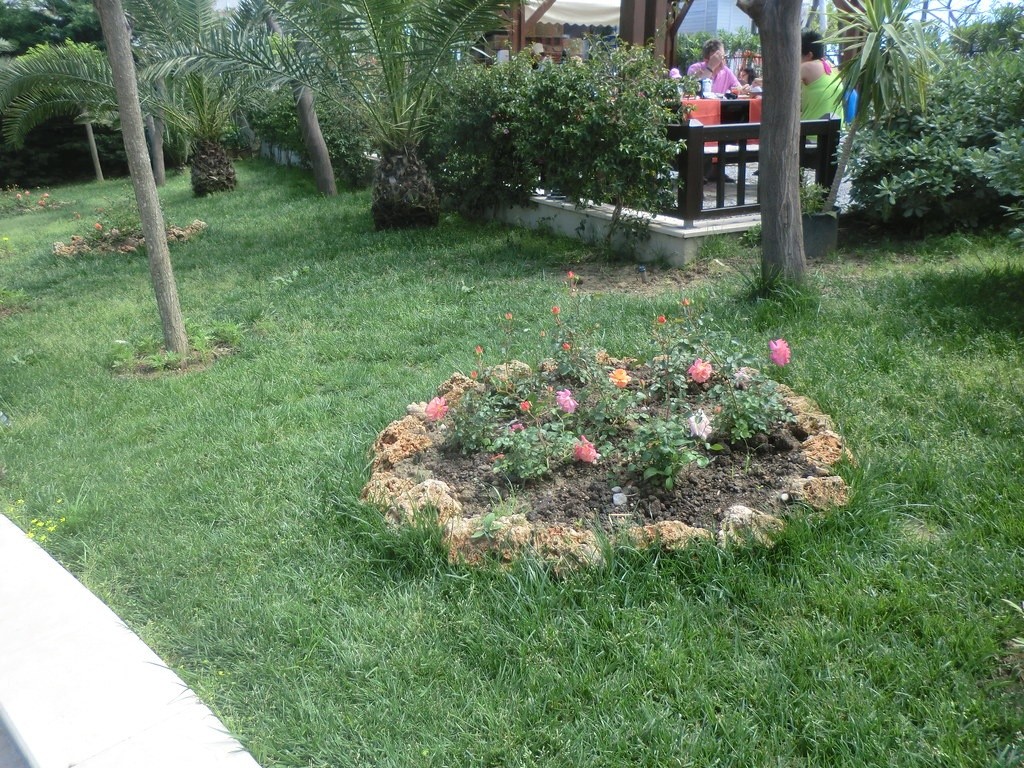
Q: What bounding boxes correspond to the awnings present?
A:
[525,0,620,26]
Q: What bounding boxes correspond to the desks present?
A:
[666,99,762,146]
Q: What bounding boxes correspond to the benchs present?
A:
[704,143,816,164]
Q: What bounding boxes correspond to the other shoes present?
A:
[725,174,735,183]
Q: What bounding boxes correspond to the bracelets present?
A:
[705,65,713,73]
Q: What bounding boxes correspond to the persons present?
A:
[749,77,762,93]
[522,41,623,75]
[735,67,758,96]
[671,69,684,96]
[839,70,863,124]
[688,39,752,182]
[750,31,845,176]
